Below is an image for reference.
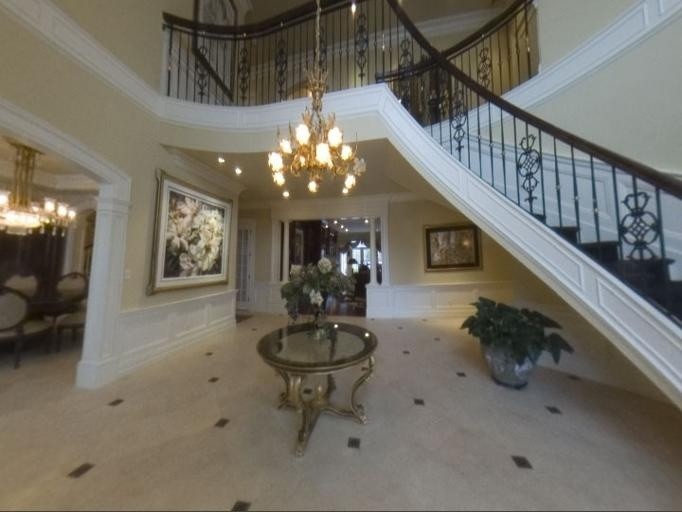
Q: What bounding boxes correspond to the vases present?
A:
[311,307,329,331]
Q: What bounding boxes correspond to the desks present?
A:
[255,323,377,456]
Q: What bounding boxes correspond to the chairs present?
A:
[0,271,88,368]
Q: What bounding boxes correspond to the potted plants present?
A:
[459,297,575,387]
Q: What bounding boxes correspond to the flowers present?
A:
[280,254,357,329]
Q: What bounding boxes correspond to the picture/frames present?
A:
[146,168,232,296]
[422,221,482,271]
[193,0,237,100]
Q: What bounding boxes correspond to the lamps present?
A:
[268,0,360,198]
[0,138,77,238]
